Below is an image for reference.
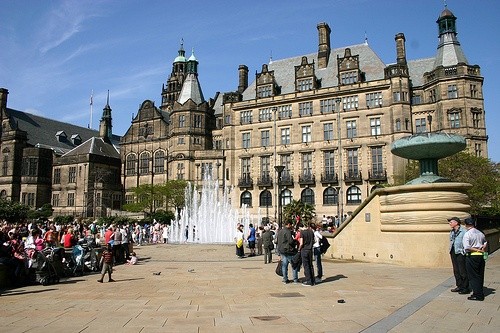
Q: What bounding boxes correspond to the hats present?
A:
[447,217,461,224]
[465,218,474,225]
[264,226,269,231]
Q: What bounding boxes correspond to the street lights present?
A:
[274,165,286,230]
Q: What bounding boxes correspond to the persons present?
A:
[447,216,487,301]
[234,214,322,286]
[185,225,188,239]
[0,219,169,283]
[319,214,348,234]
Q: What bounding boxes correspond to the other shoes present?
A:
[97,279,103,282]
[302,282,313,286]
[451,288,472,294]
[282,280,288,284]
[108,278,116,282]
[294,280,301,284]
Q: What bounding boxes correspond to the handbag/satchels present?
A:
[275,260,282,276]
[269,243,274,250]
[237,239,242,248]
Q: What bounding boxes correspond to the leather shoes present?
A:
[468,295,483,301]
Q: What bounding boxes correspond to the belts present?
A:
[465,252,483,255]
